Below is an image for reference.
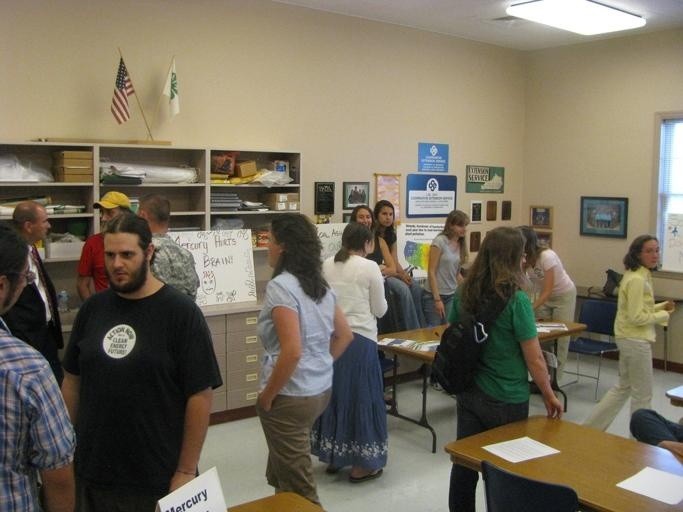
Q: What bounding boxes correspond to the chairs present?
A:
[478,459,580,512]
[567,299,625,403]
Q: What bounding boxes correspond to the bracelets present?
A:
[434,298,442,303]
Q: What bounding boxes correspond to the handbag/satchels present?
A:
[428,322,489,395]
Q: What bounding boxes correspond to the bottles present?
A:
[58,289,69,312]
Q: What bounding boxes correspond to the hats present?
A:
[93,190,132,211]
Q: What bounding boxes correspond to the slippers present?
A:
[348,468,384,482]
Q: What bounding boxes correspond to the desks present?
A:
[572,283,682,373]
[666,384,683,408]
[371,315,588,453]
[226,489,328,512]
[443,416,683,512]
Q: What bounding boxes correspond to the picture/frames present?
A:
[578,194,629,240]
[342,212,354,223]
[529,205,554,230]
[534,232,553,250]
[469,200,482,225]
[343,181,370,209]
[373,171,402,226]
[313,181,334,216]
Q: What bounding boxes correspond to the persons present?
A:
[374,200,427,330]
[589,207,618,229]
[348,186,366,205]
[61,211,222,511]
[350,205,396,333]
[0,202,77,511]
[78,191,200,303]
[516,225,577,381]
[447,227,563,512]
[629,409,683,457]
[421,210,470,399]
[579,235,676,433]
[256,213,354,506]
[310,222,389,483]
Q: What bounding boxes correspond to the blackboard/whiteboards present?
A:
[313,222,350,266]
[166,229,257,307]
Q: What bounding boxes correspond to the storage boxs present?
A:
[258,191,299,212]
[234,157,255,177]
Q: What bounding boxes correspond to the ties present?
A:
[32,247,57,324]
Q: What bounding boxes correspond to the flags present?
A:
[163,59,179,123]
[111,57,135,130]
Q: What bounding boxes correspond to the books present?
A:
[210,190,243,212]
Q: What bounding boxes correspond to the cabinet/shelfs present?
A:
[0,143,301,427]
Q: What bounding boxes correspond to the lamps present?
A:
[506,0,647,39]
